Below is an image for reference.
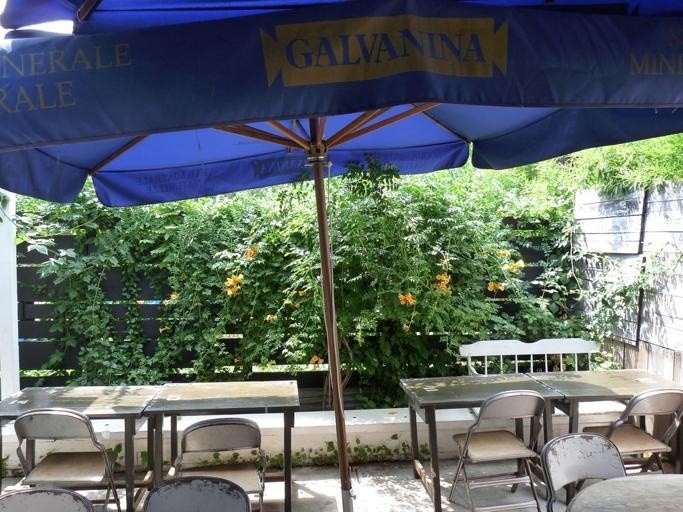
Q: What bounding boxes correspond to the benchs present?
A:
[459,339,627,418]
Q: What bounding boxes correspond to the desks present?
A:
[567,475,683,512]
[0,386,163,512]
[397,373,564,512]
[143,380,301,512]
[526,369,683,472]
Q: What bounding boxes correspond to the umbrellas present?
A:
[0,0,683,491]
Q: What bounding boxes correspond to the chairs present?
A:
[174,418,264,512]
[142,477,250,511]
[15,407,120,511]
[449,389,545,512]
[0,487,93,512]
[583,388,683,473]
[540,433,627,512]
